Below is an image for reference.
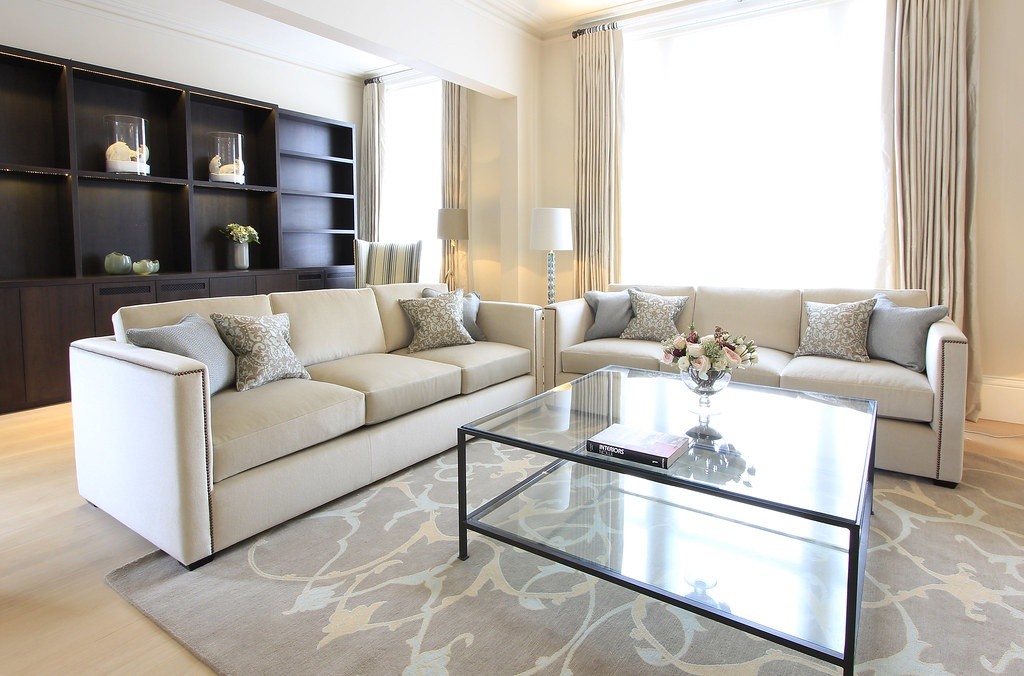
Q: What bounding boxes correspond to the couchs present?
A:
[69,283,546,565]
[543,282,972,490]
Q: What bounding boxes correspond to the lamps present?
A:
[437,206,468,288]
[530,208,574,305]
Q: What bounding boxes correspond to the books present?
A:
[586,423,690,469]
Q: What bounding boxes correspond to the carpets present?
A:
[104,448,1024,675]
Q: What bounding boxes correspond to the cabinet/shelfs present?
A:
[0,46,357,415]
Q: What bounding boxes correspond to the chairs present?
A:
[354,240,422,289]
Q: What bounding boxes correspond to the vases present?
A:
[677,364,730,422]
[232,242,252,272]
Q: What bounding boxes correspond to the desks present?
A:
[452,362,883,676]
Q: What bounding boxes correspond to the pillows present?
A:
[858,293,950,372]
[423,289,483,340]
[394,289,475,353]
[210,312,314,391]
[628,288,686,345]
[125,312,237,396]
[583,289,638,338]
[796,300,871,359]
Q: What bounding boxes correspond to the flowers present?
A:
[218,222,262,245]
[642,325,765,379]
